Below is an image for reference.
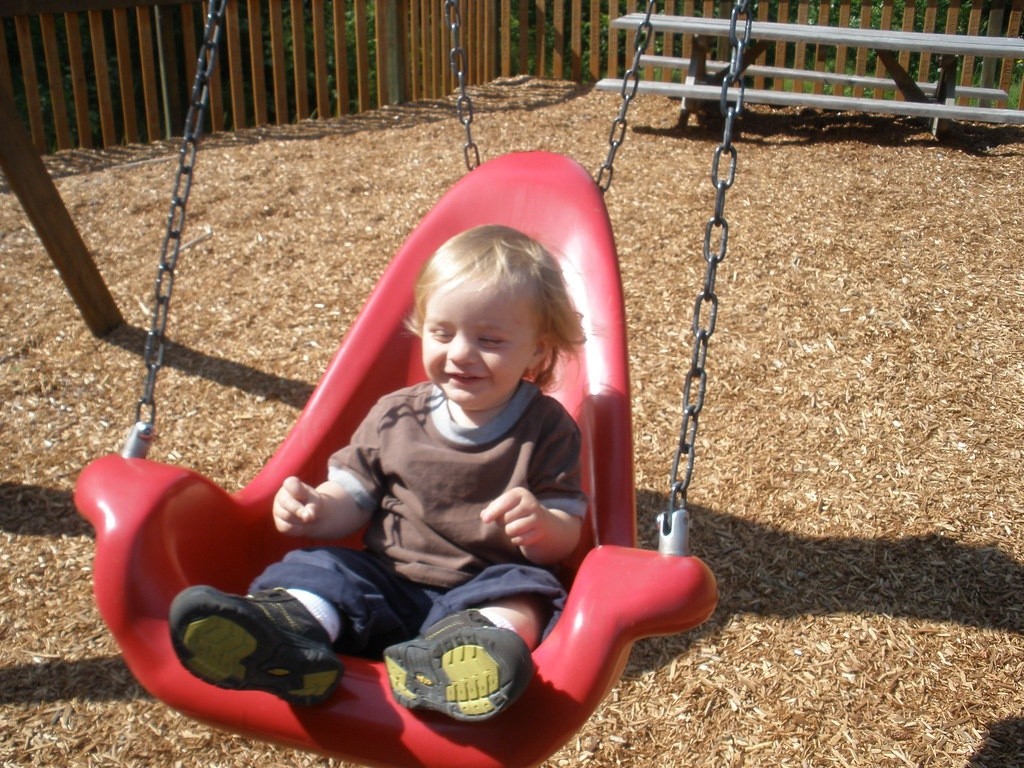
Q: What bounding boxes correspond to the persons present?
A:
[168,224,588,720]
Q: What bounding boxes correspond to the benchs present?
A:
[595,52,1024,126]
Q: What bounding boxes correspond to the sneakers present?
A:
[383,610,531,722]
[167,584,345,706]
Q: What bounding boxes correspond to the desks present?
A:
[610,11,1024,137]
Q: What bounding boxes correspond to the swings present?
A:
[69,0,770,768]
